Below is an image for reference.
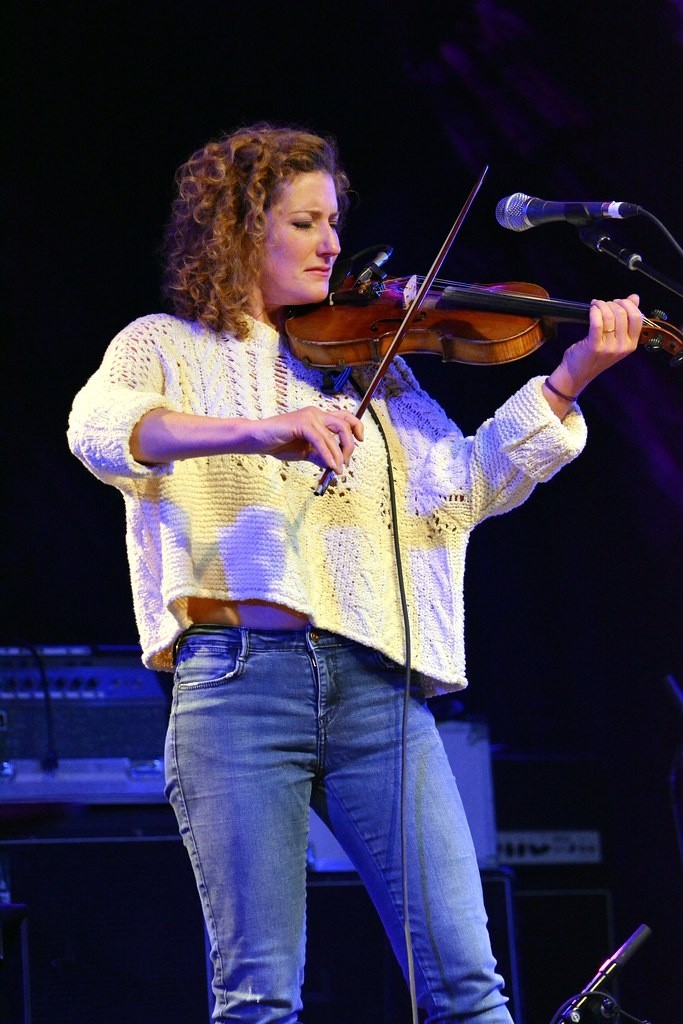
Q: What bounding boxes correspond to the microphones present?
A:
[359,249,393,282]
[496,193,640,233]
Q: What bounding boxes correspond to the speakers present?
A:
[1,803,624,1024]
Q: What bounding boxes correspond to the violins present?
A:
[285,272,682,366]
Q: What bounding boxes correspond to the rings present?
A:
[602,330,617,333]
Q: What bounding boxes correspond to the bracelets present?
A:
[541,378,579,406]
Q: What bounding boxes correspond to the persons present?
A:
[66,121,645,1024]
